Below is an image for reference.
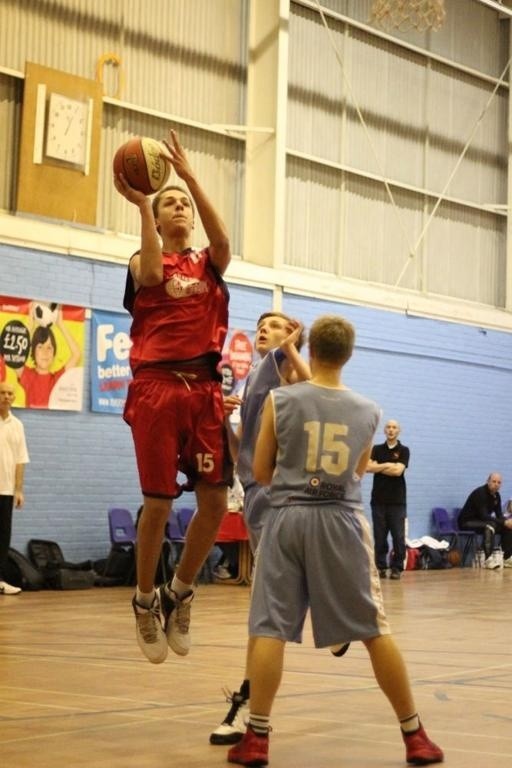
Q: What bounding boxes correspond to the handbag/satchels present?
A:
[389,544,462,571]
[27,539,95,590]
[472,546,504,570]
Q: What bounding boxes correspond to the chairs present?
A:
[431,506,512,566]
[101,507,250,584]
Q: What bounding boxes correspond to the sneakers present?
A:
[210,688,251,746]
[0,581,22,595]
[378,569,387,578]
[228,722,272,766]
[398,717,444,763]
[390,566,400,579]
[210,565,232,578]
[484,559,500,569]
[504,555,512,568]
[132,590,168,664]
[156,581,194,656]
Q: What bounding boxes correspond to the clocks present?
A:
[32,84,95,175]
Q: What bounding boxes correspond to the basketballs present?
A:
[113,137,170,195]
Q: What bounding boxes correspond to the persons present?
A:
[208,305,352,747]
[456,472,512,571]
[13,302,85,410]
[110,125,235,668]
[1,382,30,600]
[365,417,412,583]
[226,313,447,767]
